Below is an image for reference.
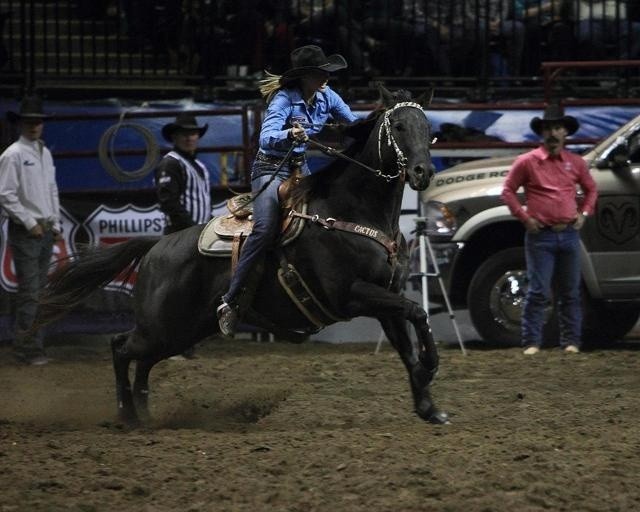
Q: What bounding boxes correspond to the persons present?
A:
[100,0,639,107]
[217,44,362,341]
[154,112,215,361]
[0,98,65,367]
[499,103,600,355]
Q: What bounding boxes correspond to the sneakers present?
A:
[15,347,48,365]
[218,299,237,337]
[565,345,579,353]
[524,347,540,354]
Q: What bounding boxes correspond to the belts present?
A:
[542,222,571,232]
[11,217,53,231]
[256,151,306,165]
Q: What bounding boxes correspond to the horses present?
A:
[14,84,450,428]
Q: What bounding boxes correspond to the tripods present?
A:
[374,188,468,357]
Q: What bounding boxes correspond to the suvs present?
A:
[416,111,639,350]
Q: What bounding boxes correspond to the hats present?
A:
[279,45,347,85]
[531,105,579,136]
[162,112,208,142]
[7,97,56,120]
